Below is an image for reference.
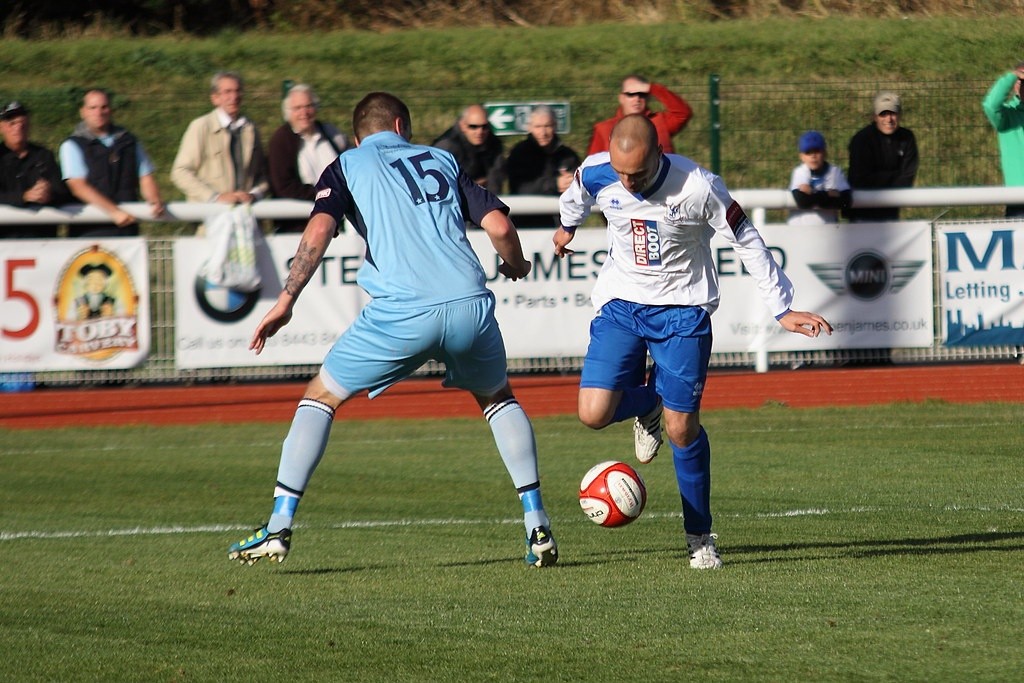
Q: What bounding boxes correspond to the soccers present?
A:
[579,461,647,528]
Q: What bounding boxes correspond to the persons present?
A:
[982,64,1024,217]
[268,83,352,233]
[790,131,853,217]
[229,91,559,567]
[553,114,833,570]
[587,75,692,228]
[430,104,504,229]
[59,88,164,236]
[506,106,581,228]
[841,93,917,222]
[169,71,274,238]
[0,100,62,238]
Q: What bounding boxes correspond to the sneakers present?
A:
[633,394,664,464]
[227,523,292,567]
[686,533,723,570]
[524,525,559,569]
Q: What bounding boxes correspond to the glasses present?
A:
[463,122,489,129]
[621,91,650,98]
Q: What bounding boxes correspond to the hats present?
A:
[799,132,825,152]
[872,92,902,115]
[0,100,29,121]
